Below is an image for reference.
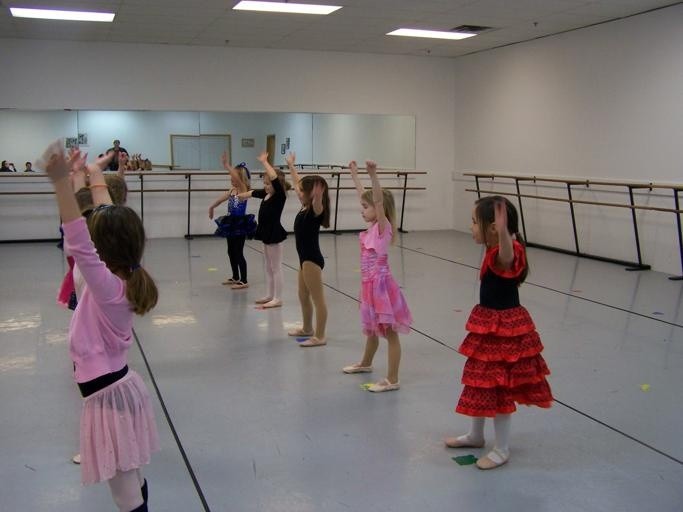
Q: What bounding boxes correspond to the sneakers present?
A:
[261,300,282,308]
[368,376,401,392]
[231,281,249,289]
[446,433,486,449]
[343,362,374,373]
[299,336,328,347]
[288,327,314,336]
[222,278,240,284]
[474,448,510,469]
[255,297,273,303]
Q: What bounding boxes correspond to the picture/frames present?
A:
[241,137,255,147]
[285,138,290,149]
[281,144,285,154]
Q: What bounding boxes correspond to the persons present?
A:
[35,138,160,511]
[282,150,331,350]
[233,150,288,307]
[0,139,153,466]
[206,149,257,291]
[443,193,554,471]
[340,159,414,393]
